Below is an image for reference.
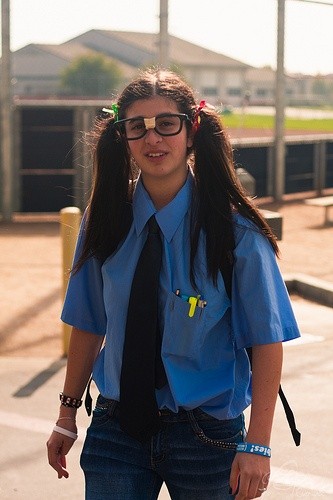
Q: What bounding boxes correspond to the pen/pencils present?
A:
[174,286,208,318]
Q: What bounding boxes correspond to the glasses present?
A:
[113,113,188,140]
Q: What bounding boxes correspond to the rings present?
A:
[257,488,267,493]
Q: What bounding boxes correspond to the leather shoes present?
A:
[118,222,164,425]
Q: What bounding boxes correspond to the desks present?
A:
[304,196,333,229]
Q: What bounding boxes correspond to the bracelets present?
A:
[59,392,83,408]
[58,417,75,420]
[53,426,78,440]
[235,442,271,458]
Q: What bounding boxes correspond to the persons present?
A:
[47,68,301,500]
